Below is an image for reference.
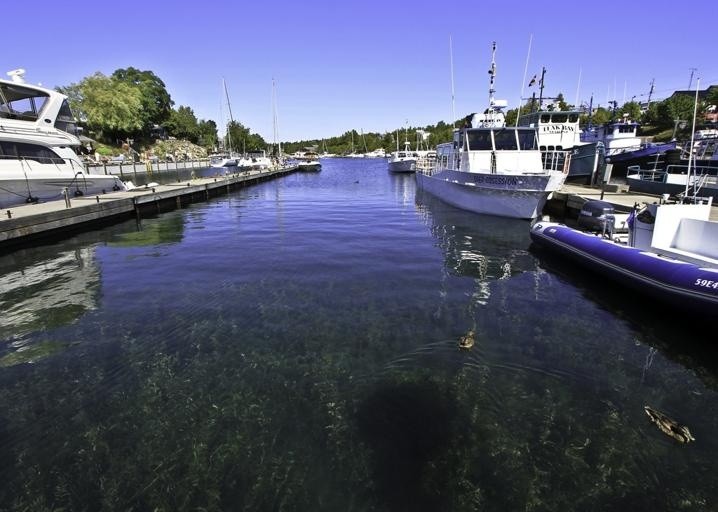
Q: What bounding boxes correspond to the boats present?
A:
[0,69,126,207]
[414,37,574,224]
[387,132,422,174]
[507,64,610,188]
[299,160,321,172]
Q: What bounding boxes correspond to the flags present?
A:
[528,74,538,87]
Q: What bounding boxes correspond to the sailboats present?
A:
[237,75,283,170]
[211,78,240,168]
